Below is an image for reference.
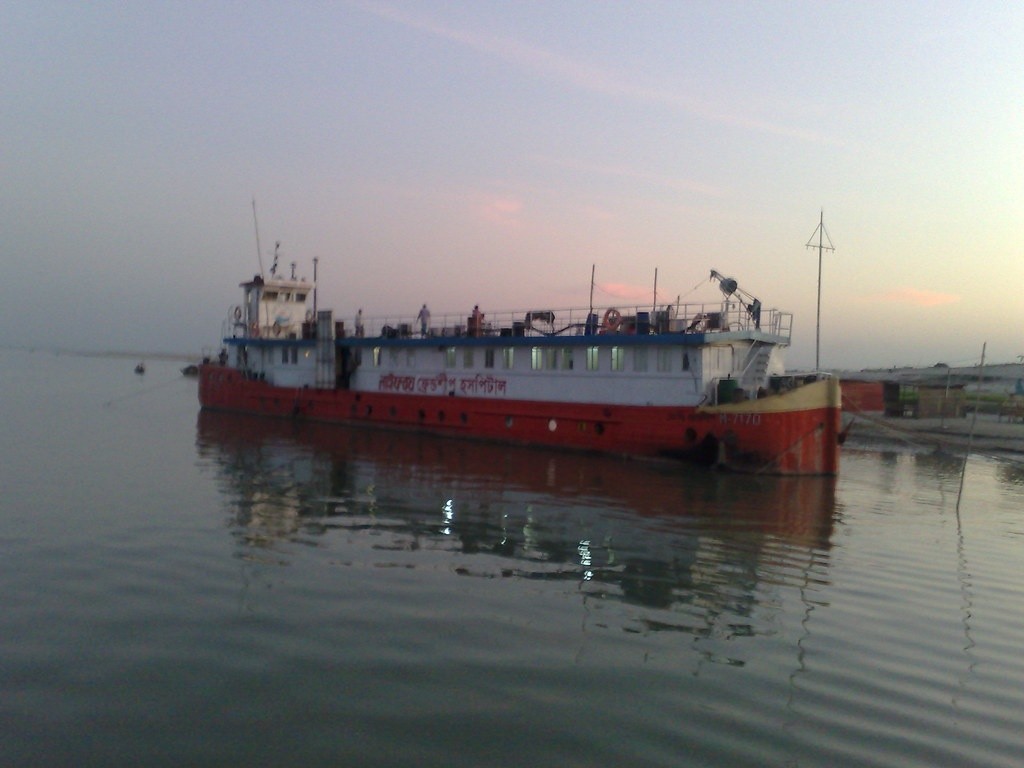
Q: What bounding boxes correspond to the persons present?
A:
[355,309,364,338]
[416,304,431,338]
[472,305,491,337]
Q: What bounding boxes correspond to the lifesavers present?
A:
[603,307,621,330]
[233,305,242,321]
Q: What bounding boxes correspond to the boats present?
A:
[194,237,841,479]
